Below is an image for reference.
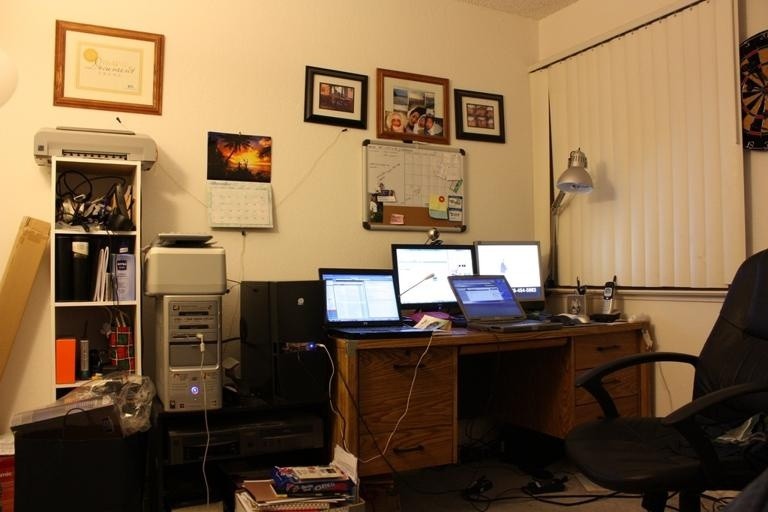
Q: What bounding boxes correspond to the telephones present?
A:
[590,275,621,323]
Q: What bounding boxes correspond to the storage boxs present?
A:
[234,487,368,511]
[8,427,169,510]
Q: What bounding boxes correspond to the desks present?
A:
[327,316,657,489]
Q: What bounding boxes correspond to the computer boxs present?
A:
[240,279,327,414]
[154,296,224,414]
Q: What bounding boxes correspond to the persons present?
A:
[387,112,408,132]
[404,107,424,133]
[412,114,427,135]
[424,114,442,136]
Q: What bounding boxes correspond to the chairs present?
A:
[562,246,767,510]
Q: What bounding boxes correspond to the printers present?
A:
[33,126,159,172]
[142,233,228,296]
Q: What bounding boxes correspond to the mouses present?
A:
[573,315,591,324]
[551,313,574,326]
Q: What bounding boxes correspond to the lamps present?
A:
[548,149,596,289]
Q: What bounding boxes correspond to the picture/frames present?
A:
[374,67,451,147]
[51,18,167,117]
[303,63,369,132]
[453,88,508,144]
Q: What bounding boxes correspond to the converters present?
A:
[467,479,494,495]
[527,477,566,494]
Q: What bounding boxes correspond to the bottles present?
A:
[108,241,136,302]
[55,235,90,301]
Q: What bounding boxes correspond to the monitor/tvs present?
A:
[391,244,477,325]
[472,240,547,318]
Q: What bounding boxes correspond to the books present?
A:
[92,245,110,303]
[237,443,360,512]
[413,314,453,335]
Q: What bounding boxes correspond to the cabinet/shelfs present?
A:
[155,398,330,510]
[49,154,143,406]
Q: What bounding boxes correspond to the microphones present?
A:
[398,273,437,298]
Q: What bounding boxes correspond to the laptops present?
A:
[448,275,564,333]
[318,267,434,340]
